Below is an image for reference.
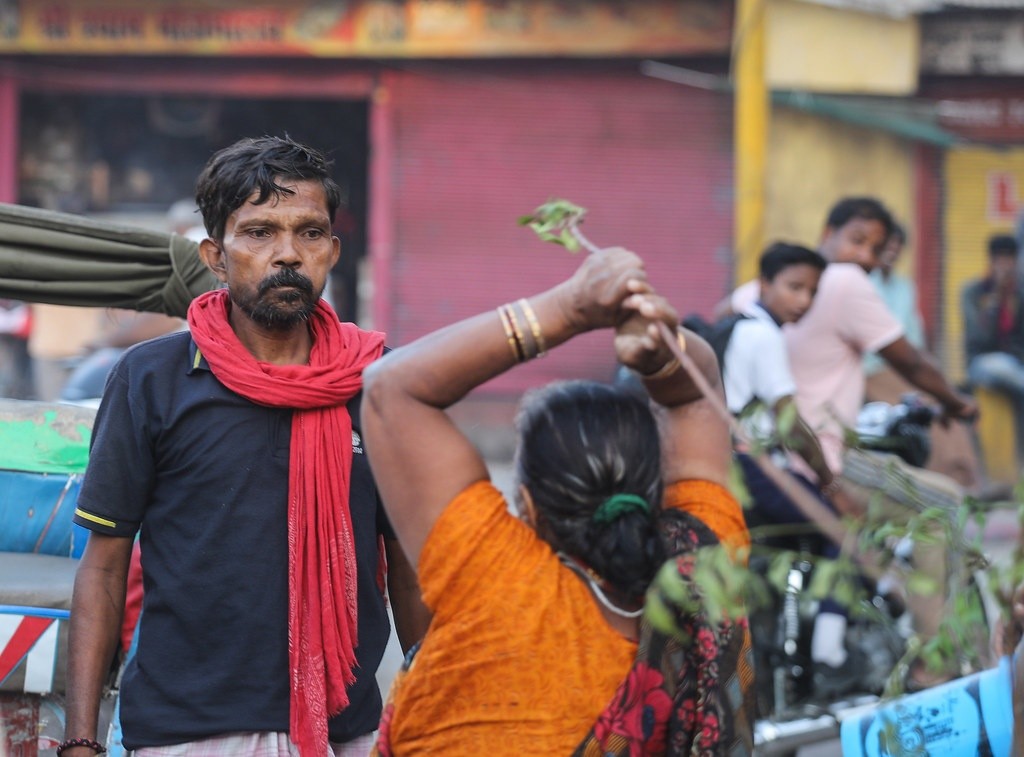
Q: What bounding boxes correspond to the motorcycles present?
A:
[730,379,974,727]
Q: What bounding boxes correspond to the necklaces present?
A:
[556,551,647,620]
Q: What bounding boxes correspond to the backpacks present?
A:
[682,311,762,419]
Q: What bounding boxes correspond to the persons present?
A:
[56,134,430,756]
[963,232,1024,479]
[866,225,926,404]
[715,241,865,684]
[716,196,979,690]
[359,244,755,757]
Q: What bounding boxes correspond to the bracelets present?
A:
[628,331,686,381]
[57,737,106,756]
[498,296,547,363]
[822,477,841,498]
